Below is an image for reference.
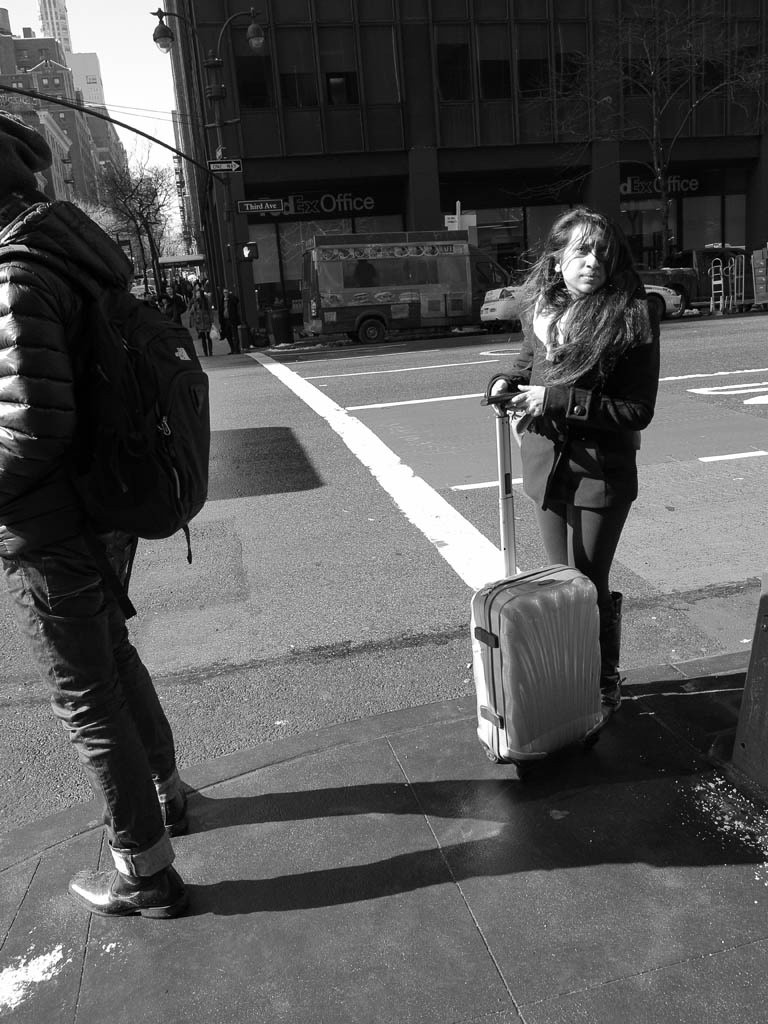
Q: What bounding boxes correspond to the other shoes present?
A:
[228,351,241,355]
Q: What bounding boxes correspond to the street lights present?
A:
[151,5,269,353]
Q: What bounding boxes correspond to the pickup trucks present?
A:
[637,246,756,321]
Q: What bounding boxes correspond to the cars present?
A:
[129,277,158,302]
[480,285,525,332]
[644,285,684,319]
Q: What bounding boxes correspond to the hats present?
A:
[0,109,53,193]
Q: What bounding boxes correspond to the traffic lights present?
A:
[241,242,258,260]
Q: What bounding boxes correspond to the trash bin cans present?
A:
[269,308,294,345]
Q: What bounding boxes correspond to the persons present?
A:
[487,207,661,757]
[1,114,210,919]
[167,276,241,362]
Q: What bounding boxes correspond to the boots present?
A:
[601,590,623,718]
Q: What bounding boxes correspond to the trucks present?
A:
[302,230,513,346]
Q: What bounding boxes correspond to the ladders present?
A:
[708,257,725,315]
[724,257,735,306]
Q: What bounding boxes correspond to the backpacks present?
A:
[0,236,210,539]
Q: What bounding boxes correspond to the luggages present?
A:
[469,394,605,763]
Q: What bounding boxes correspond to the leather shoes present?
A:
[158,784,189,836]
[68,865,187,919]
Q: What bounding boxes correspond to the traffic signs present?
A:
[207,159,243,173]
